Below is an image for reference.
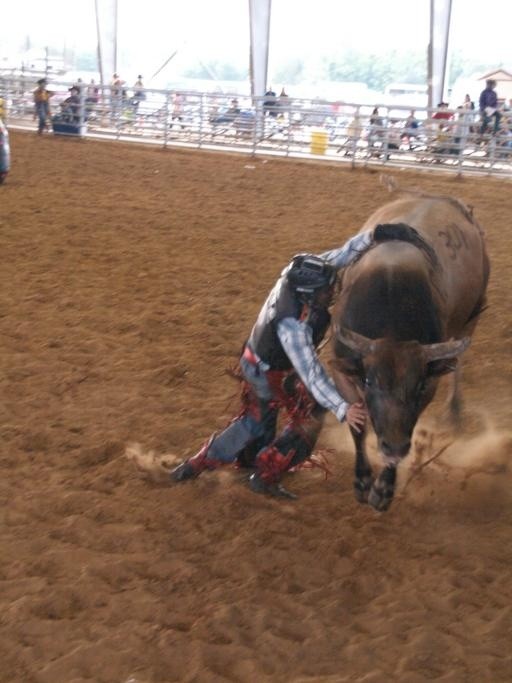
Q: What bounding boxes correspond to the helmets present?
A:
[286,253,343,310]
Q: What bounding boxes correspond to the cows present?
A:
[318,193,492,513]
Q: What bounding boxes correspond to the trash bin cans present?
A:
[310,130,327,154]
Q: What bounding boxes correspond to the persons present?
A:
[33,77,55,134]
[133,73,147,101]
[110,72,121,98]
[262,84,277,115]
[215,98,240,122]
[60,85,84,122]
[167,220,426,498]
[338,74,511,164]
[87,78,99,104]
[278,86,288,117]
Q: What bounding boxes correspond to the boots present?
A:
[171,430,228,483]
[249,430,313,501]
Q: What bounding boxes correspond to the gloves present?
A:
[373,222,418,244]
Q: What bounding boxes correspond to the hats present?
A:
[69,86,80,93]
[438,103,449,108]
[112,74,143,79]
[37,79,48,85]
